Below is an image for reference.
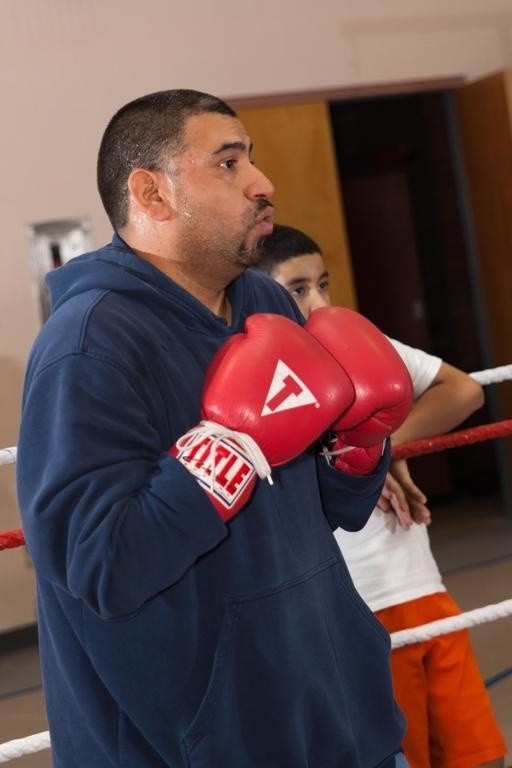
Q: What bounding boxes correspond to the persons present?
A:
[16,88,414,766]
[251,223,507,768]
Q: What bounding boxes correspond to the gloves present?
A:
[168,312,356,524]
[304,305,414,475]
